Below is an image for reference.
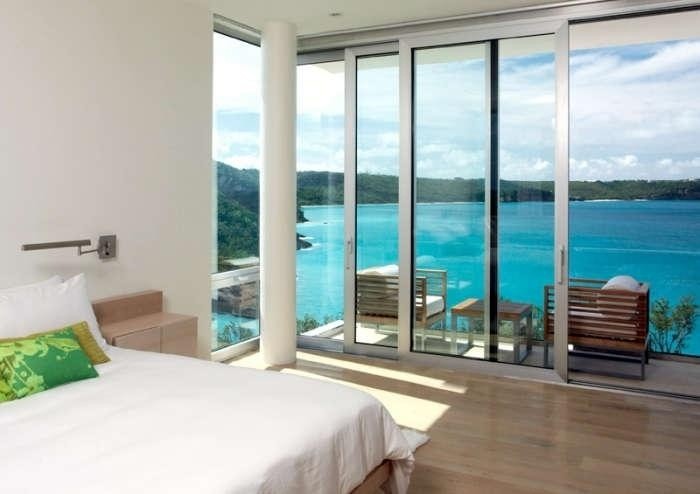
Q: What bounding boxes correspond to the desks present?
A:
[450,298,533,364]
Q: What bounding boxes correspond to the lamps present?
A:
[21,235,116,258]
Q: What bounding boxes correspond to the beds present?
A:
[1,348,414,494]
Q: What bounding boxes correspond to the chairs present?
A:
[357,264,447,352]
[542,279,651,381]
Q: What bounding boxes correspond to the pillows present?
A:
[597,274,641,322]
[0,273,108,405]
[360,264,399,299]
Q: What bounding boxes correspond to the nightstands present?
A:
[92,289,198,358]
